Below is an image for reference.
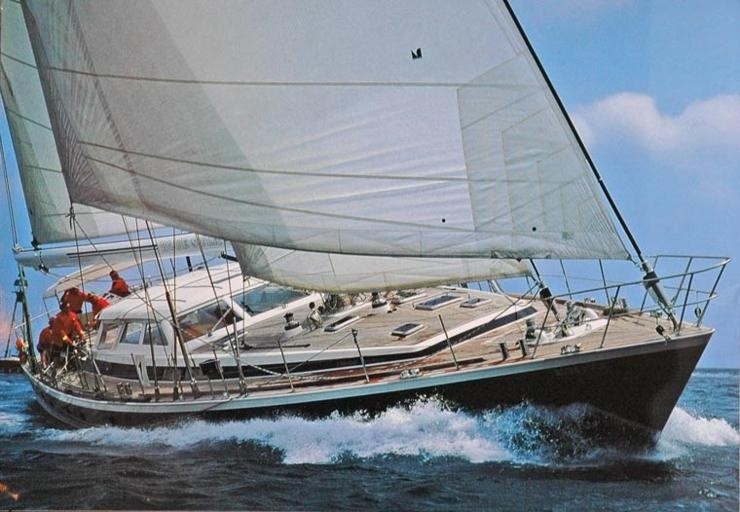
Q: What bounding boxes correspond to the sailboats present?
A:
[1,0,735,451]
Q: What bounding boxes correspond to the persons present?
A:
[37,270,131,370]
